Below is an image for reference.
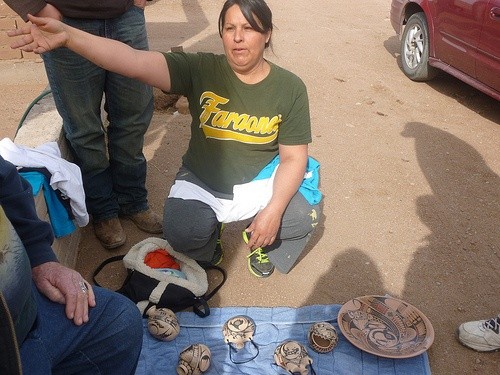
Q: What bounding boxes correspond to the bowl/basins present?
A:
[336,295,434,358]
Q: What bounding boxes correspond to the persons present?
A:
[0,156,142,375]
[7,0,322,278]
[1,0,164,248]
[456,313,500,352]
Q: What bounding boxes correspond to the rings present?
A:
[80,282,89,292]
[252,240,256,245]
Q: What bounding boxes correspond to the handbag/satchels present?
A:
[90,236,227,319]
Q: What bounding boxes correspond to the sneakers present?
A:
[457,315,500,353]
[128,205,164,234]
[91,215,126,249]
[208,222,224,267]
[244,222,276,279]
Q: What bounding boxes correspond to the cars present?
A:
[391,0,500,102]
